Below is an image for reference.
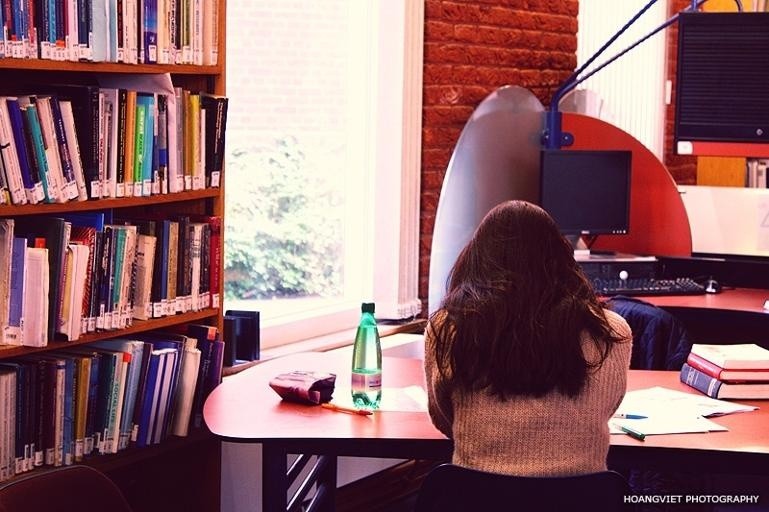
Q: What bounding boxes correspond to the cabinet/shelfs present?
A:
[0,0,226,512]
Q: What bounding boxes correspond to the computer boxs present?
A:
[573,255,666,280]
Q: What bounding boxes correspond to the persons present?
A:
[421,197,635,478]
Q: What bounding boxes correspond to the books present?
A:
[747,160,768,189]
[692,342,769,370]
[0,0,229,485]
[686,353,769,381]
[680,363,769,401]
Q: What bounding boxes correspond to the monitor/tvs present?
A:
[539,149,632,256]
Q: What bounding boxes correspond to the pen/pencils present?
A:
[613,414,648,440]
[321,402,372,415]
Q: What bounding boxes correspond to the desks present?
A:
[203,286,769,512]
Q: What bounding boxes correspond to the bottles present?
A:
[350,302,383,411]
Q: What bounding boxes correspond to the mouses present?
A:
[704,279,720,293]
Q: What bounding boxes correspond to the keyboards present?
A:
[588,277,705,295]
